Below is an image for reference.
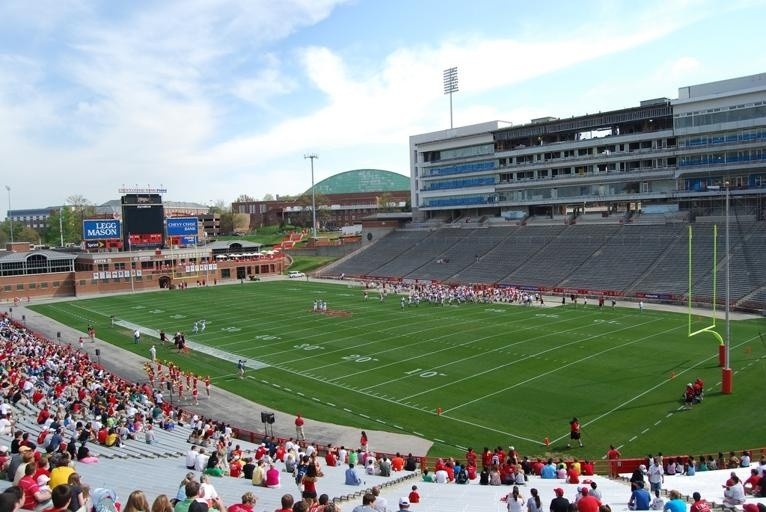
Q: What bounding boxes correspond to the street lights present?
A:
[442,65,461,131]
[5,185,14,243]
[304,152,319,236]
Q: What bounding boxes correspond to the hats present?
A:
[687,383,693,388]
[37,474,50,485]
[638,463,648,473]
[578,485,584,492]
[260,443,266,447]
[509,445,515,451]
[34,452,46,459]
[553,487,564,495]
[188,499,209,512]
[235,456,240,461]
[581,487,589,494]
[398,496,411,506]
[19,445,32,454]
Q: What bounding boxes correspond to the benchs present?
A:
[267,452,765,511]
[1,366,267,512]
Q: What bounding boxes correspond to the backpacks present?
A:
[458,470,467,484]
